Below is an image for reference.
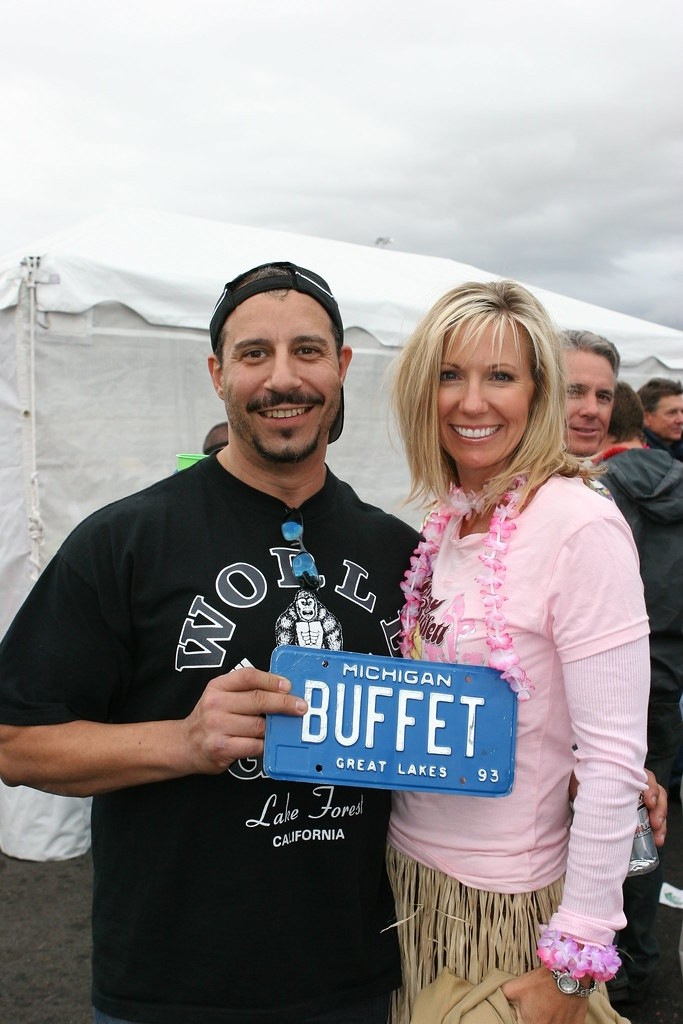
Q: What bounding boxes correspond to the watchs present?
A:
[552,970,597,998]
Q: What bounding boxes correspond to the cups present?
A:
[177,454,210,473]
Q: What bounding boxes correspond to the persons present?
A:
[556,329,683,1024]
[174,423,229,475]
[0,261,669,1024]
[391,280,651,1024]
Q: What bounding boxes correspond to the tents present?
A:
[0,178,681,863]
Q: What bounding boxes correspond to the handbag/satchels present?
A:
[411,968,633,1024]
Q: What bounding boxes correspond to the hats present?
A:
[210,262,345,444]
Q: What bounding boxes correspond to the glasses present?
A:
[280,507,319,592]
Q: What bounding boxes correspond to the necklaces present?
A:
[399,474,535,702]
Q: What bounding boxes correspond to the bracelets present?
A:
[537,924,622,982]
